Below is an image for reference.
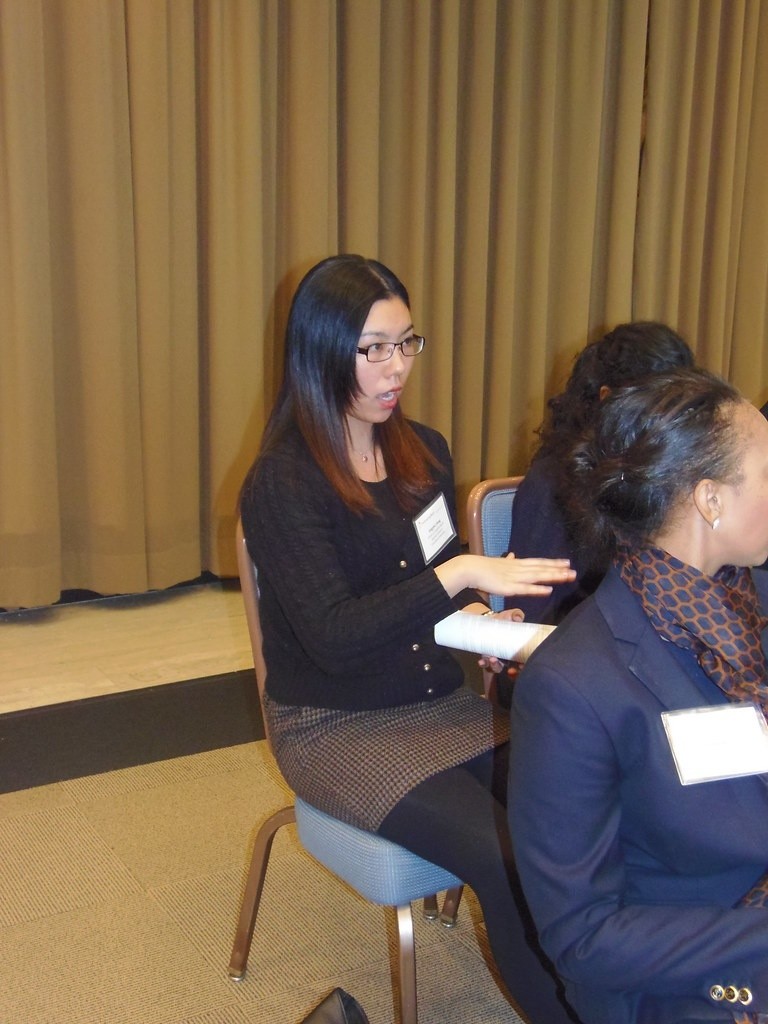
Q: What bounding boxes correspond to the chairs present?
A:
[225,475,534,1024]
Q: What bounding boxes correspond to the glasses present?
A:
[354,331,424,362]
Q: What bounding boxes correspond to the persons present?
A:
[236,253,576,1024]
[499,319,694,712]
[508,359,767,1024]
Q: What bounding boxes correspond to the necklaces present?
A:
[349,441,375,461]
[483,611,495,616]
